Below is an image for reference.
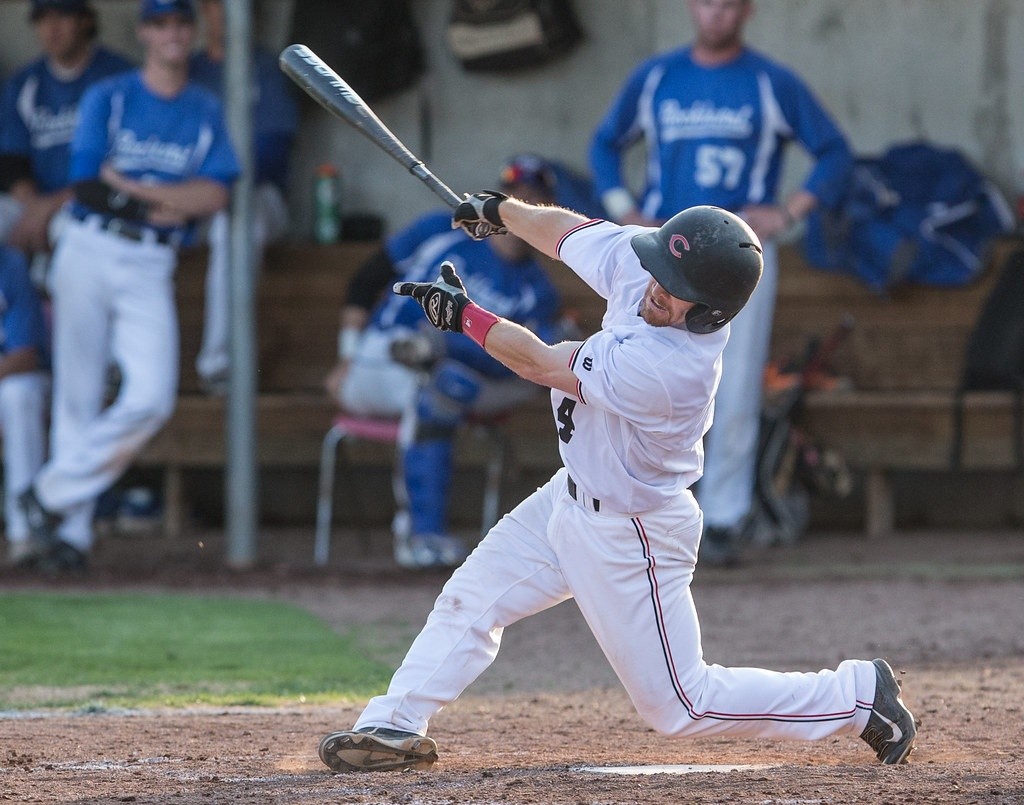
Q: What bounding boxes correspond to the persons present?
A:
[319,187,916,770]
[1,0,246,575]
[588,0,859,563]
[326,156,568,569]
[190,0,301,392]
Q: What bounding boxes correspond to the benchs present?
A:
[142,213,1024,547]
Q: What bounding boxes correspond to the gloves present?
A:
[451,189,510,241]
[392,260,472,333]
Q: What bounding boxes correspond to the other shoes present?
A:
[14,487,61,548]
[700,525,737,562]
[396,537,462,570]
[12,540,90,583]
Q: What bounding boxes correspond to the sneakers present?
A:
[858,658,923,765]
[318,727,439,772]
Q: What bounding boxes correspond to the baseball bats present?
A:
[277,42,492,239]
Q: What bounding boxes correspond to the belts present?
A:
[82,210,170,245]
[567,473,600,513]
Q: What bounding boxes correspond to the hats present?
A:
[138,0,195,20]
[496,153,552,192]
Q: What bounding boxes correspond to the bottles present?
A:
[310,164,345,247]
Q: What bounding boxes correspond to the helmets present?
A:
[631,204,762,333]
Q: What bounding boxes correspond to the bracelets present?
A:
[601,187,634,218]
[778,204,794,226]
[462,304,500,348]
[338,328,357,360]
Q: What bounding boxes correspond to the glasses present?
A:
[498,164,557,190]
